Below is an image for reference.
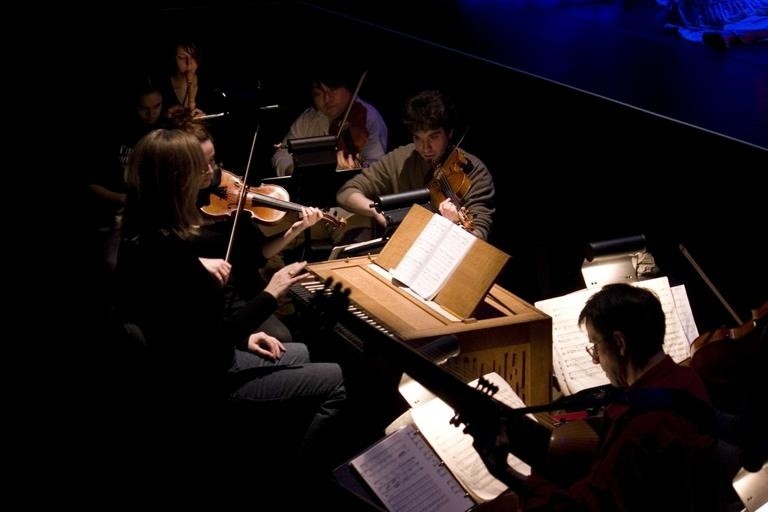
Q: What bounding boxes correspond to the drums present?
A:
[328,101,369,169]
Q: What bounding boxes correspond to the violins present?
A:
[198,167,347,232]
[429,147,476,232]
[677,303,768,415]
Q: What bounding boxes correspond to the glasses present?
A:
[585,337,608,360]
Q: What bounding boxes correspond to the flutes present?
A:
[187,70,196,117]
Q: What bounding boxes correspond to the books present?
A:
[351,423,476,511]
[393,213,479,301]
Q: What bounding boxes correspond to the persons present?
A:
[82,31,358,510]
[336,91,498,255]
[270,48,387,209]
[476,283,721,510]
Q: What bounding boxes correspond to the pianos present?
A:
[287,204,553,410]
